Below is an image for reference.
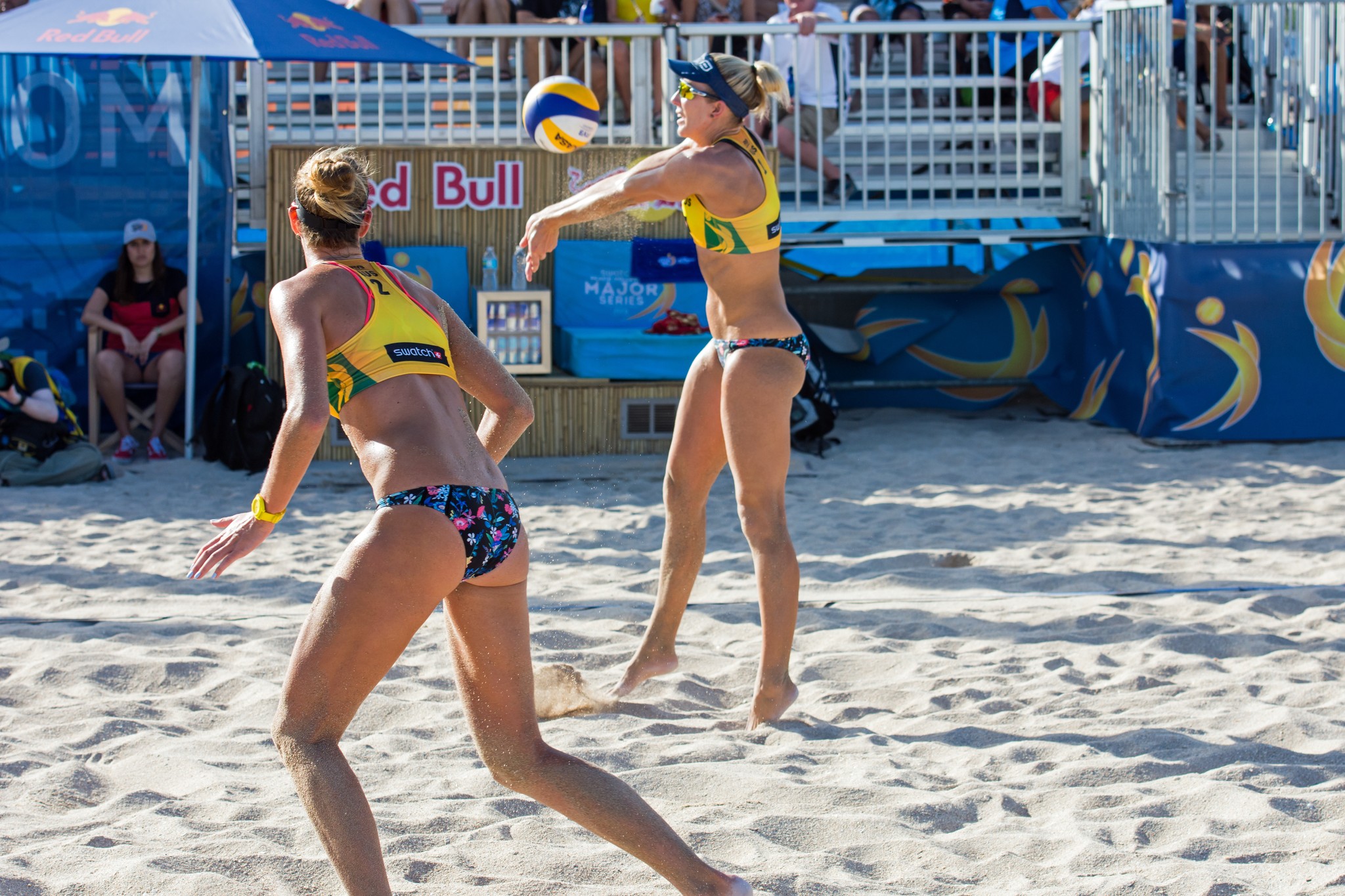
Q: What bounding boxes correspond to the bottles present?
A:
[482,245,498,292]
[511,246,527,290]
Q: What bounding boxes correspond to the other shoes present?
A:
[456,68,470,80]
[408,71,420,80]
[1203,138,1224,152]
[500,69,511,80]
[823,173,856,203]
[1218,117,1247,128]
[350,75,369,82]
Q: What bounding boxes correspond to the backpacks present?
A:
[218,359,284,473]
[790,362,842,454]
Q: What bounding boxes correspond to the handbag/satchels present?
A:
[961,52,996,105]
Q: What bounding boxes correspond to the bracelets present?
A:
[14,395,26,407]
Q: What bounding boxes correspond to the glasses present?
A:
[678,79,719,105]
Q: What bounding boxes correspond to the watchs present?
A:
[251,493,286,523]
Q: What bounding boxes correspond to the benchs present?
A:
[555,238,712,378]
[237,0,1296,195]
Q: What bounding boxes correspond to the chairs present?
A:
[84,324,183,454]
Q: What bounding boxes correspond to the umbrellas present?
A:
[0,0,481,466]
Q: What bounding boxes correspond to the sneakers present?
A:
[113,436,138,463]
[146,438,168,463]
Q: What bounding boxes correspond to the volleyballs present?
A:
[521,75,600,155]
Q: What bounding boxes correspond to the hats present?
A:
[668,51,749,119]
[123,219,156,243]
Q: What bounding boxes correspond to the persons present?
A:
[184,145,750,896]
[0,337,112,487]
[80,220,202,464]
[519,53,808,730]
[348,0,1262,218]
